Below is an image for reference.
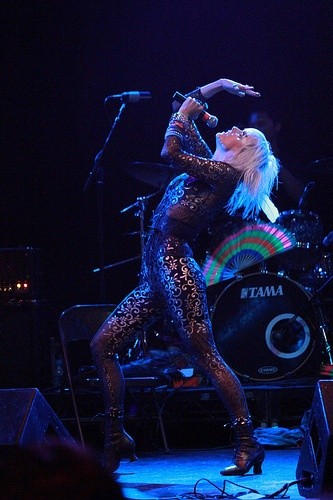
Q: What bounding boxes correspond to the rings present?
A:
[232,83,237,88]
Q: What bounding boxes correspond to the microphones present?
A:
[323,231,333,246]
[173,92,218,128]
[277,329,289,339]
[107,92,152,102]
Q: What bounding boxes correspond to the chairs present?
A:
[58,303,170,456]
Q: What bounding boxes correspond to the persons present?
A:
[0,443,124,500]
[89,79,280,476]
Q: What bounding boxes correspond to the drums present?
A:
[273,208,323,260]
[210,271,322,382]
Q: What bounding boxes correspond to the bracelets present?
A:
[165,113,189,142]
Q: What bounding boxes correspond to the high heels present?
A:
[219,420,265,477]
[102,417,138,472]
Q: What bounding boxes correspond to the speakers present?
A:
[0,388,76,446]
[0,298,56,393]
[295,380,333,498]
